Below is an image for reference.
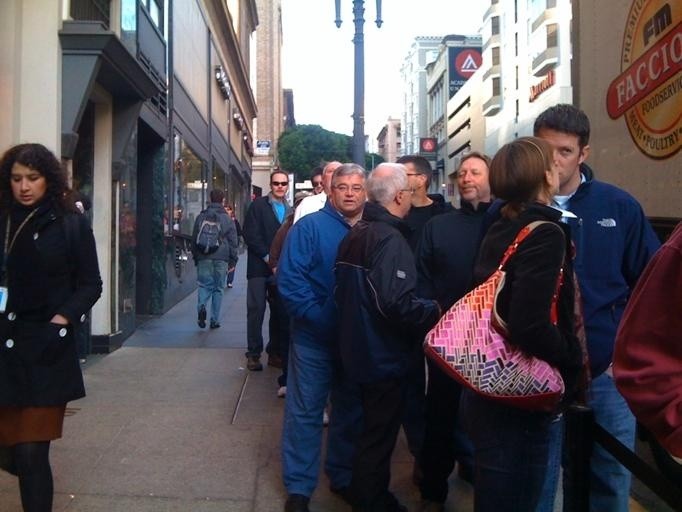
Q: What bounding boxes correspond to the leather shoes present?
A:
[198,307,207,328]
[457,467,473,483]
[247,356,263,371]
[330,485,348,499]
[267,353,285,369]
[284,493,309,511]
[422,499,445,511]
[210,321,220,329]
[412,463,423,485]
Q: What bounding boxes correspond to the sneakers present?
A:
[277,385,287,397]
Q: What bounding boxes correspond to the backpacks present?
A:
[195,209,228,254]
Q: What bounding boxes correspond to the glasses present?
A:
[271,182,289,186]
[312,182,322,187]
[403,186,417,194]
[331,185,361,193]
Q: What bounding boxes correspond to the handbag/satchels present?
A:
[422,221,566,414]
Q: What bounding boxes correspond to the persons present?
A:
[242,149,494,512]
[117,201,136,314]
[192,188,238,329]
[473,105,681,512]
[0,143,103,512]
[164,203,195,284]
[223,205,241,289]
